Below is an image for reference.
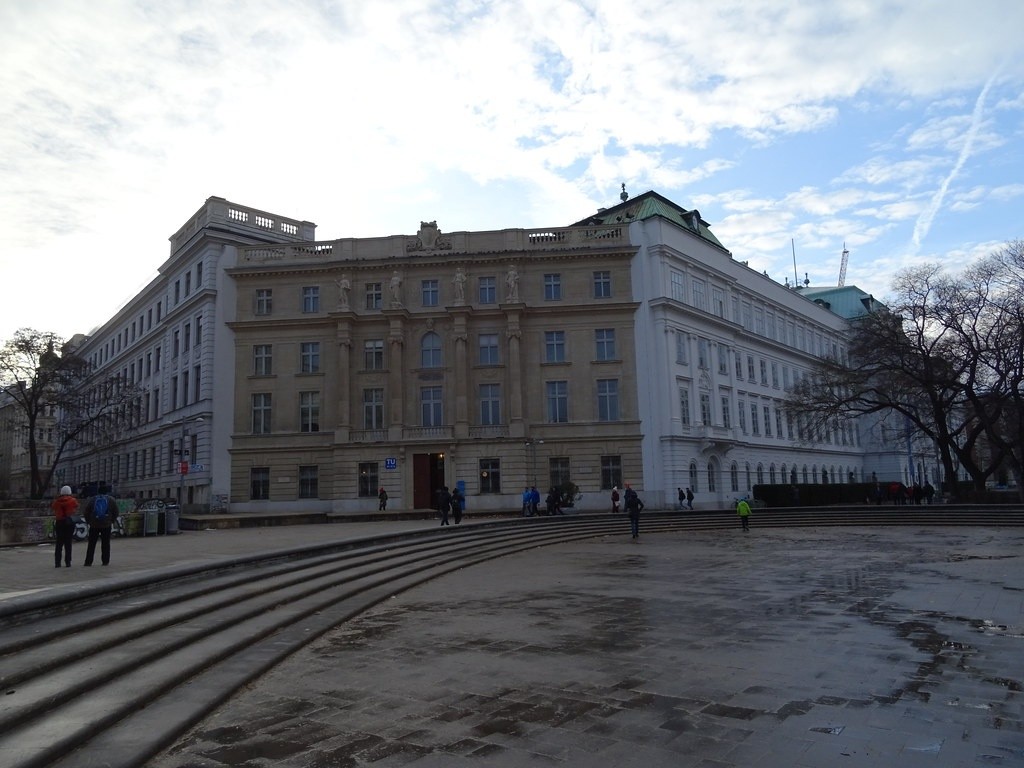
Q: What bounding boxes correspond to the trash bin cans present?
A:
[164,504,180,536]
[143,508,158,537]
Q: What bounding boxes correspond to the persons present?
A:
[79,483,120,566]
[737,498,753,532]
[551,487,563,516]
[437,486,453,526]
[611,486,620,514]
[527,486,543,518]
[378,487,387,511]
[51,486,78,566]
[450,486,466,525]
[874,478,937,505]
[623,482,635,513]
[677,487,688,511]
[685,487,694,511]
[545,492,556,516]
[626,491,644,539]
[520,487,532,518]
